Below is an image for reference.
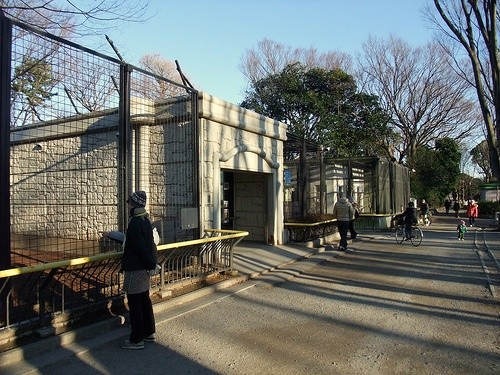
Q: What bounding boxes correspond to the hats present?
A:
[460,220,465,225]
[126,191,146,208]
[348,196,353,202]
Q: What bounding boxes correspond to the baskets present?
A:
[395,217,405,225]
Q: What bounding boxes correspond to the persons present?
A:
[444,199,450,214]
[466,200,478,228]
[397,201,419,241]
[419,199,429,226]
[333,192,354,250]
[347,196,358,240]
[457,220,467,241]
[452,200,460,218]
[99,191,160,351]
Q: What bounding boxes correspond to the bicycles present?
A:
[418,209,430,226]
[395,218,423,247]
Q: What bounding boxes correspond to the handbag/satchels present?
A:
[355,210,359,219]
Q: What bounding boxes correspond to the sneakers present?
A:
[144,333,156,342]
[120,339,144,349]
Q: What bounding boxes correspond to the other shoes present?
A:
[405,238,410,241]
[411,236,415,238]
[458,238,461,241]
[470,225,472,228]
[351,232,357,239]
[462,239,464,241]
[472,224,474,226]
[339,246,345,251]
